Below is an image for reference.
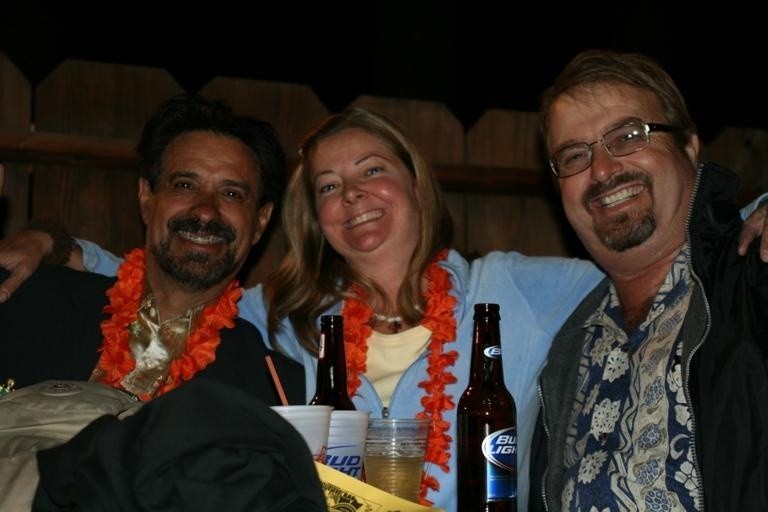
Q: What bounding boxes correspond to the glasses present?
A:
[549,121,668,178]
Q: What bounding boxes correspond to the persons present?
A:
[1,360,329,512]
[524,44,768,511]
[0,104,768,512]
[0,93,310,405]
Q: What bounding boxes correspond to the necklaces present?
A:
[368,304,419,335]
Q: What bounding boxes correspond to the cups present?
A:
[269,405,431,506]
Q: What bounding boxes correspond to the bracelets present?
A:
[50,228,74,268]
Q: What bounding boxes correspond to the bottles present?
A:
[455,303,520,511]
[308,315,359,411]
